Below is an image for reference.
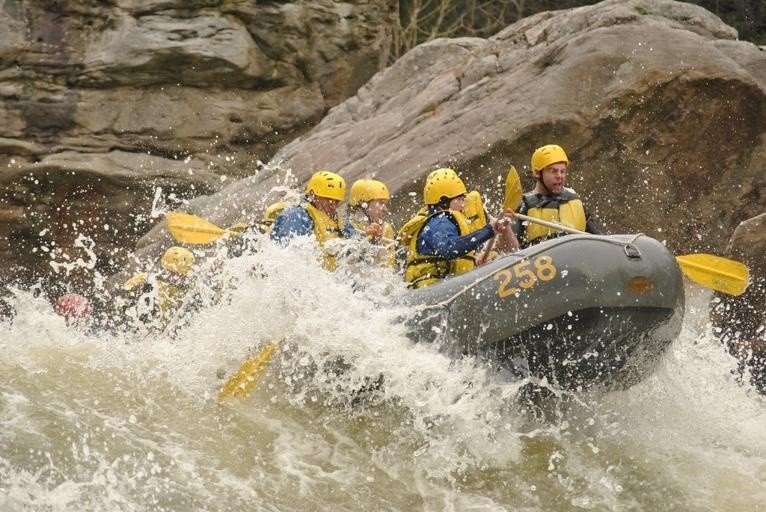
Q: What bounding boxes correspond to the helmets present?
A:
[531,145,569,172]
[266,203,292,232]
[423,168,467,205]
[160,247,195,273]
[306,170,346,201]
[349,179,390,206]
[56,294,89,318]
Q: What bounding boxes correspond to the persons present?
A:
[56,169,511,332]
[500,144,607,256]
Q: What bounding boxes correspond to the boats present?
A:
[321,233,686,404]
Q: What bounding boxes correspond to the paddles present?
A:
[482,166,525,269]
[166,209,247,246]
[218,231,379,403]
[511,213,751,296]
